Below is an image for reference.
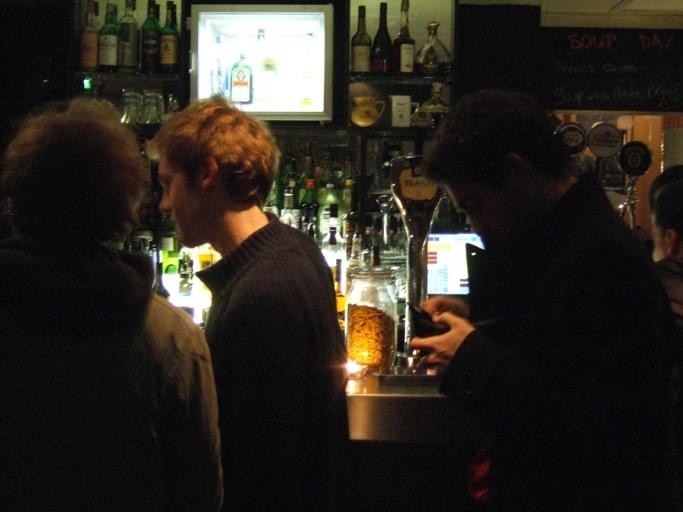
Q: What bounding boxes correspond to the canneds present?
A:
[344,268,397,367]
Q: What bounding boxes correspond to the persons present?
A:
[0,98,224,512]
[145,96,354,512]
[646,164,683,326]
[408,87,683,512]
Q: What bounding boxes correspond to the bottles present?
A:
[80,76,452,129]
[262,139,405,373]
[79,1,452,77]
[122,217,213,299]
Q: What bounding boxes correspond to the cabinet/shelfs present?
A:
[81,72,450,234]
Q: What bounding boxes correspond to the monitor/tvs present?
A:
[190,4,337,123]
[420,231,486,298]
